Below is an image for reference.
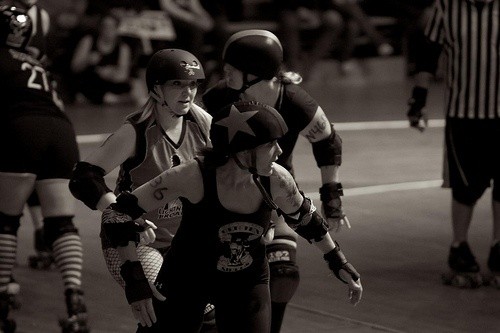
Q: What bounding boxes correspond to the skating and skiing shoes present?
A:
[59,289,90,333]
[0,280,23,333]
[28,228,55,272]
[487,241,500,290]
[439,239,483,290]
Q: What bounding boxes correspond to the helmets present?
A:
[145,49,206,91]
[222,29,284,81]
[209,100,288,155]
[0,6,33,52]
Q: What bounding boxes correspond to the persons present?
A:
[100,101,363,333]
[194,28,351,333]
[405,0,500,289]
[34,0,421,107]
[0,12,91,333]
[24,188,55,268]
[69,49,212,294]
